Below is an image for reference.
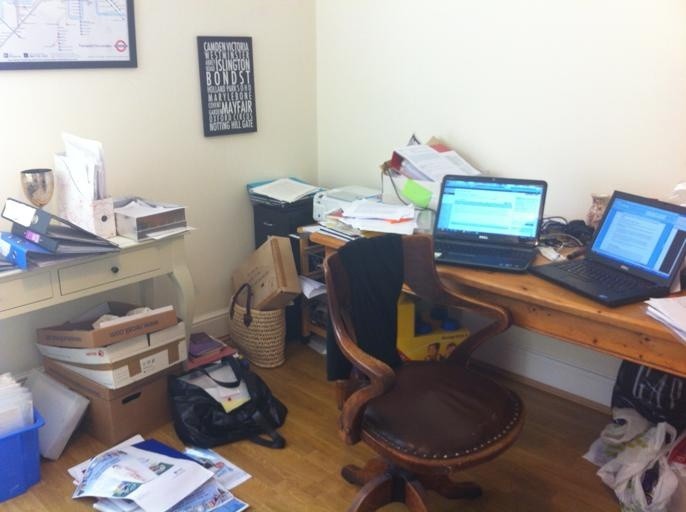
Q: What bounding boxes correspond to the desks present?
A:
[1,234,194,358]
[310,220,686,380]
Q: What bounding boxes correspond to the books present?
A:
[317,196,417,245]
[645,295,685,346]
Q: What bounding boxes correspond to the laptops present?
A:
[529,190,686,306]
[430,174,548,272]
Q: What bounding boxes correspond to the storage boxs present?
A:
[32,300,189,448]
[381,172,442,212]
[0,405,46,504]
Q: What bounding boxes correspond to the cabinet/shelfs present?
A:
[253,199,329,346]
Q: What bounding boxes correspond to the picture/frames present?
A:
[196,36,257,137]
[0,0,138,69]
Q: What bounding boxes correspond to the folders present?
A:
[1,197,121,271]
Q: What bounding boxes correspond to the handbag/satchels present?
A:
[227,281,287,366]
[167,356,289,450]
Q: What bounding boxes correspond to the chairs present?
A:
[324,231,526,511]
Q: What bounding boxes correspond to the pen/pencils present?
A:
[567,250,585,260]
